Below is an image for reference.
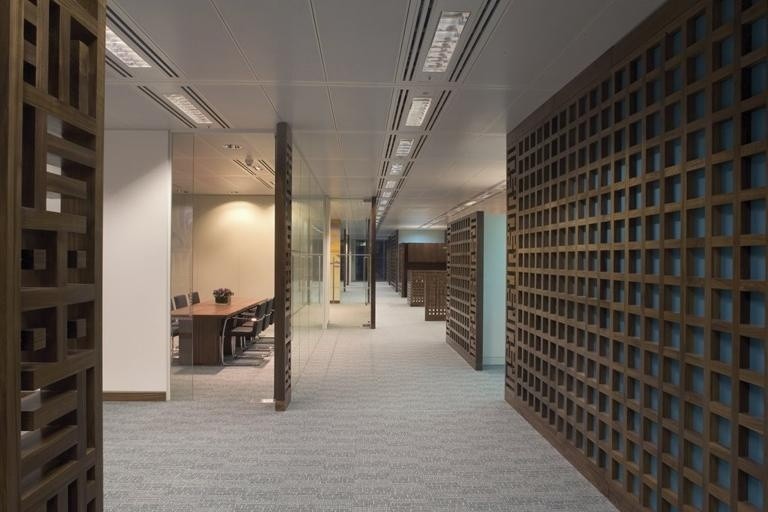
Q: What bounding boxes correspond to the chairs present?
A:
[170,291,274,367]
